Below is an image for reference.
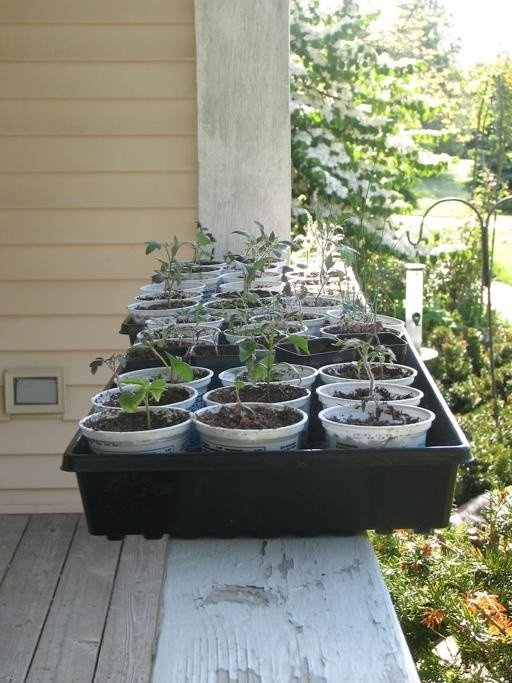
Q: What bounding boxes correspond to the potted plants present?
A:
[79,211,436,453]
